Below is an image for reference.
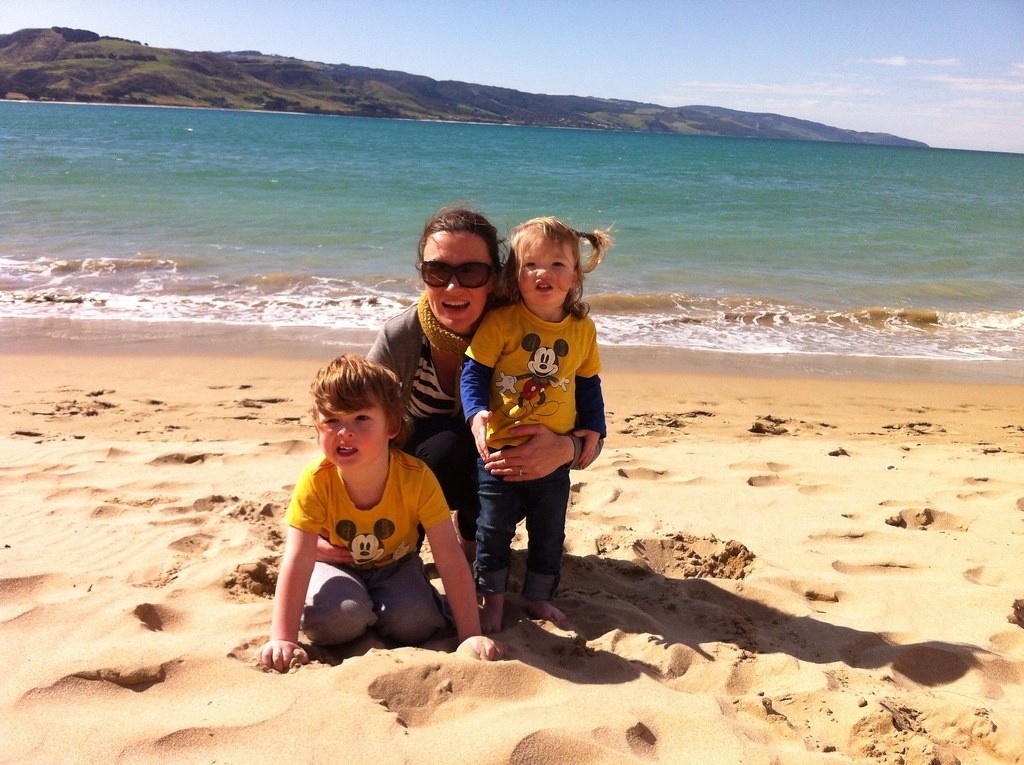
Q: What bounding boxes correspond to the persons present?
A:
[315,209,607,590]
[256,355,508,673]
[460,217,612,632]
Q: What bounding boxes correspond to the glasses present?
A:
[420,260,495,289]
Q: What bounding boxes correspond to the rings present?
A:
[520,466,523,476]
